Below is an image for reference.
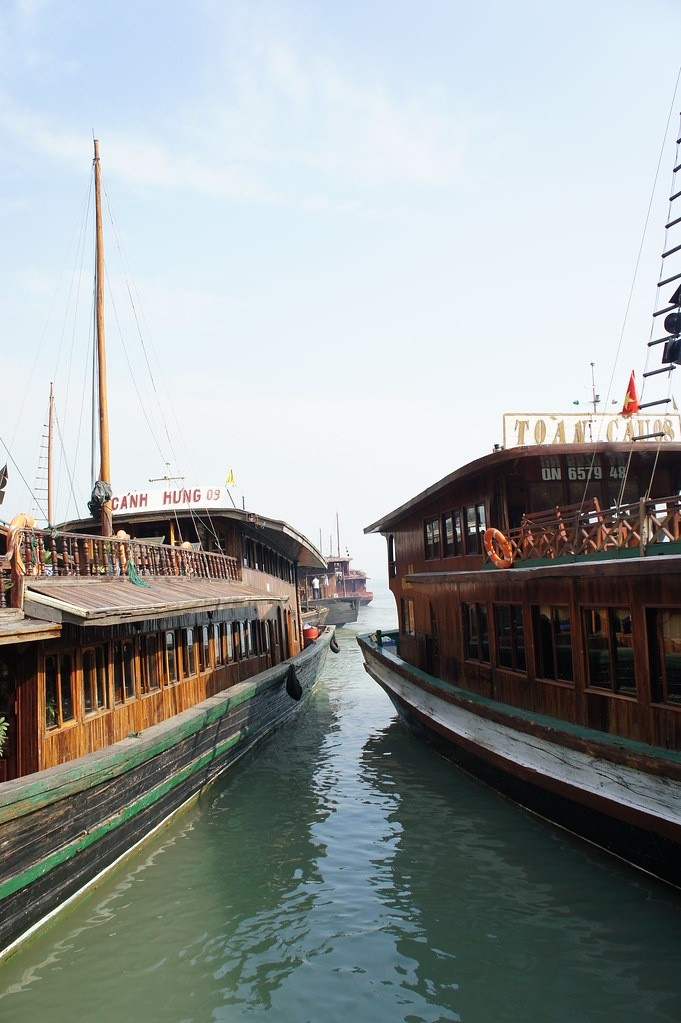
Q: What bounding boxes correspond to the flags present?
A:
[227,469,233,483]
[621,369,638,415]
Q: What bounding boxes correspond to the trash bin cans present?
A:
[303,626,318,648]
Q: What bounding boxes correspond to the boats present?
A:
[352,62,681,905]
[0,129,339,963]
[293,524,374,626]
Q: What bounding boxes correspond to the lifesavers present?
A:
[483,527,513,569]
[6,513,44,577]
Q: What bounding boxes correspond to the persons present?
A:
[311,576,319,599]
